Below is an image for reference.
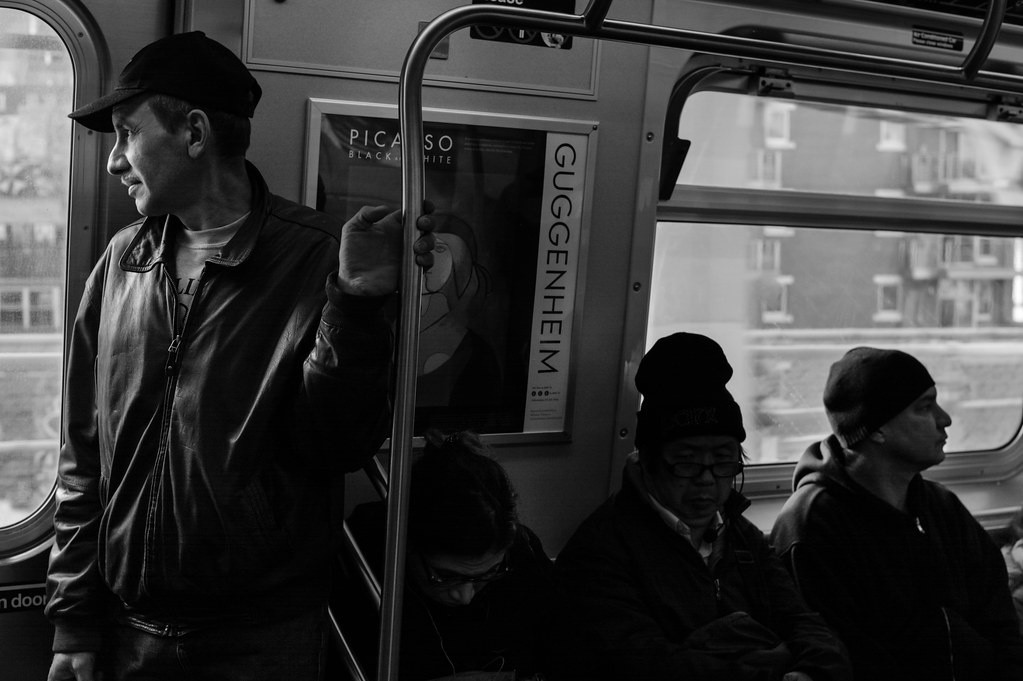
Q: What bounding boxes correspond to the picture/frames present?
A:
[302,98,601,447]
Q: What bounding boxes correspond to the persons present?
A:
[555,332,853,681]
[343,433,579,680]
[44,30,436,681]
[768,352,1023,681]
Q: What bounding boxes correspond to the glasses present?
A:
[664,461,745,479]
[419,554,510,586]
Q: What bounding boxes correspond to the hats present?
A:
[634,333,746,448]
[824,348,935,450]
[69,31,261,133]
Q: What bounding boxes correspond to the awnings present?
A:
[943,263,1014,282]
